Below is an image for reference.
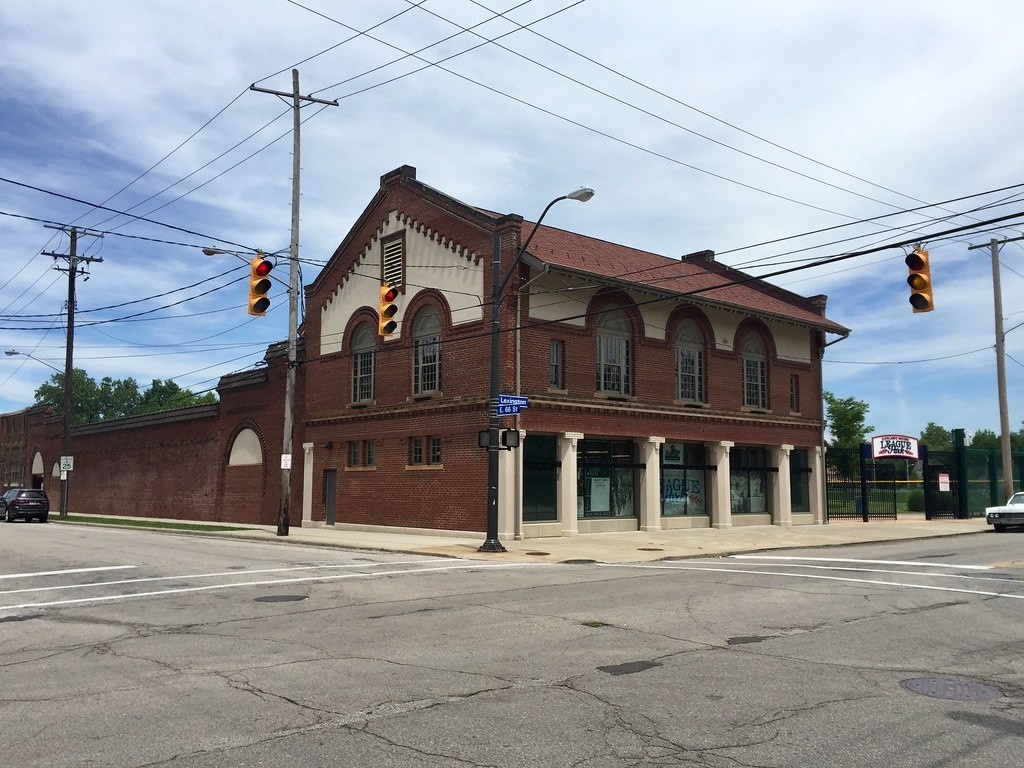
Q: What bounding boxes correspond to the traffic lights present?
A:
[378,286,399,336]
[248,257,273,316]
[905,250,935,314]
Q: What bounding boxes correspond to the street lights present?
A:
[202,245,293,291]
[5,349,67,377]
[487,185,595,297]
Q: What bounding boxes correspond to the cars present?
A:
[0,488,50,523]
[985,492,1024,532]
